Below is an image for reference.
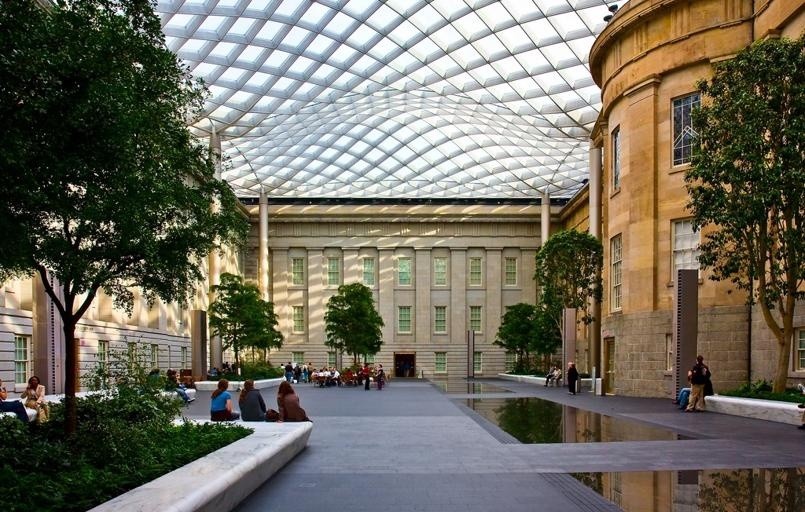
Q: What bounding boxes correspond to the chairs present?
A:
[311,376,328,388]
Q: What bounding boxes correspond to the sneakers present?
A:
[673,399,702,413]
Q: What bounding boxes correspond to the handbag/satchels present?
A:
[265,408,279,422]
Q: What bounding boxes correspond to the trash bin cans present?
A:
[575,376,581,393]
[207,370,220,377]
[180,369,191,376]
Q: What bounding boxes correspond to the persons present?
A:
[206,360,237,378]
[19,375,50,423]
[0,377,8,400]
[396,359,414,377]
[275,380,314,423]
[543,364,555,386]
[147,367,196,402]
[549,365,562,387]
[279,360,384,392]
[671,370,714,410]
[684,354,709,412]
[208,378,240,422]
[797,384,805,430]
[566,361,579,396]
[238,379,268,421]
